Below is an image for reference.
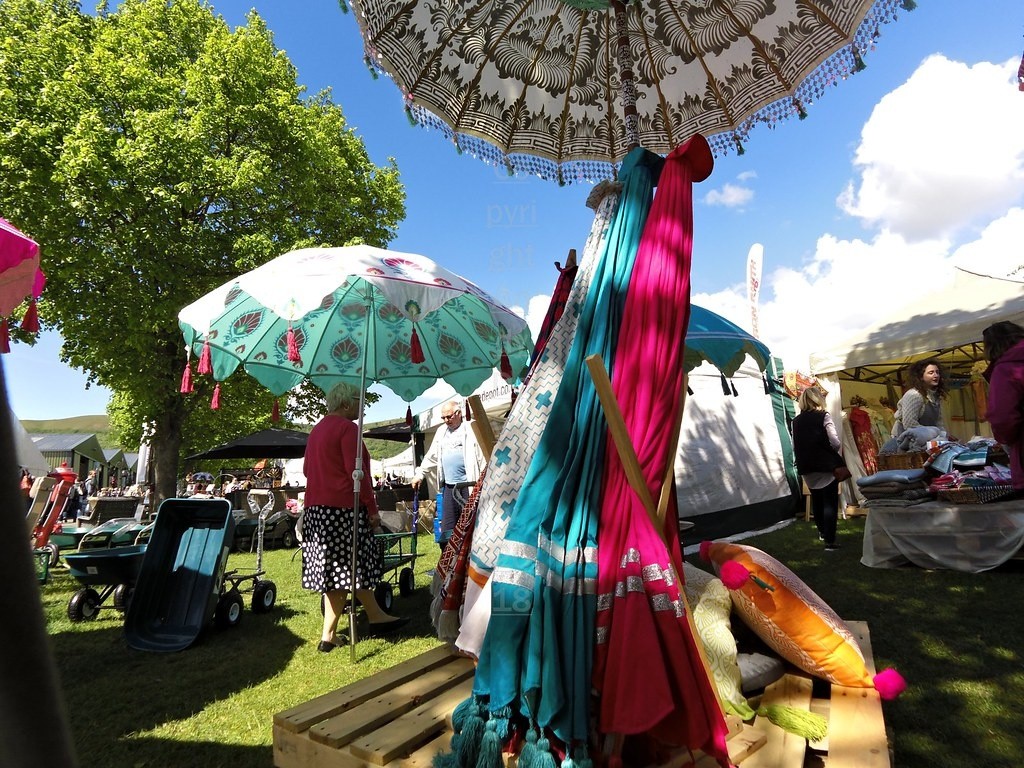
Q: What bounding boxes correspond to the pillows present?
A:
[681,562,827,743]
[698,541,907,701]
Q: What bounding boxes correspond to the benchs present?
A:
[75,498,139,527]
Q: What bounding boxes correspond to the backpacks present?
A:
[77,479,91,496]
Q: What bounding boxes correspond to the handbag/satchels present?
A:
[832,466,852,482]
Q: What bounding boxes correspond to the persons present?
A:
[890,359,946,439]
[978,320,1024,489]
[21,464,291,524]
[791,388,846,551]
[411,401,487,552]
[301,382,410,653]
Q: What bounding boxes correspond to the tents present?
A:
[809,266,1024,505]
[674,353,797,555]
[281,445,414,490]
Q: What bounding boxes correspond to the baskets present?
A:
[875,452,928,471]
[939,485,1012,505]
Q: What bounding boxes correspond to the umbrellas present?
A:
[783,369,828,403]
[342,0,913,186]
[685,303,774,396]
[430,134,728,768]
[0,218,47,355]
[178,244,533,651]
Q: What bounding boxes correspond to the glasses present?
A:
[441,409,459,420]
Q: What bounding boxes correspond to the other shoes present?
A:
[369,617,410,634]
[317,635,347,653]
[819,532,824,541]
[428,568,436,576]
[824,544,842,552]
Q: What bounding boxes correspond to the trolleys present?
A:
[33,526,92,568]
[320,482,420,617]
[62,520,155,623]
[122,488,277,653]
